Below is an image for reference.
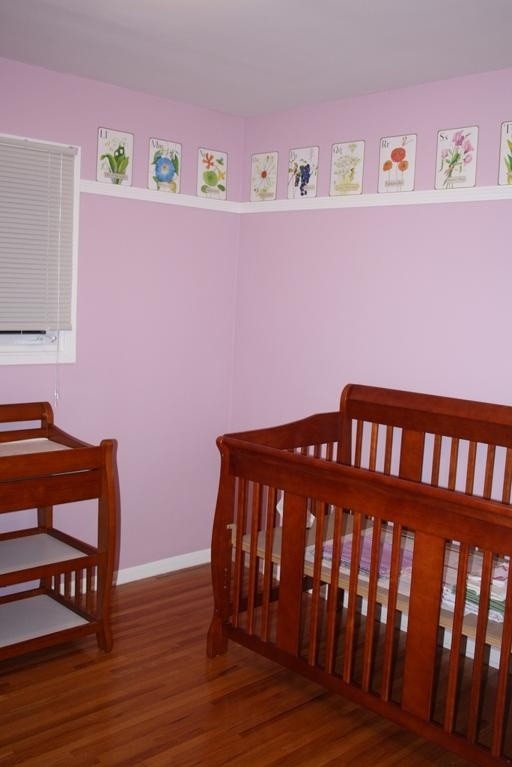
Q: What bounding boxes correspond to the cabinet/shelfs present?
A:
[2,400,122,666]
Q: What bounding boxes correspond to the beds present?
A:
[203,382,512,765]
